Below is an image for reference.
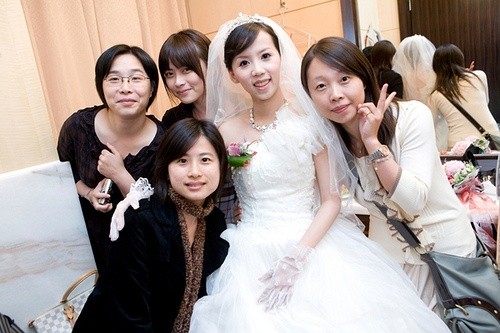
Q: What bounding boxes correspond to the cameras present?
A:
[98,179,113,205]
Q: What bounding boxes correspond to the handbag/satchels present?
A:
[422,232,500,333]
[485,130,500,150]
[28,269,98,333]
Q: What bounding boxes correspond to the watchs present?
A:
[369,145,391,161]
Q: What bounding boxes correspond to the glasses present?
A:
[103,74,151,87]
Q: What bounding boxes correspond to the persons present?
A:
[358,34,499,153]
[109,12,452,331]
[58,44,166,275]
[70,118,228,333]
[301,37,499,332]
[158,29,245,227]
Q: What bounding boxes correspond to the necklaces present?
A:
[249,99,288,132]
